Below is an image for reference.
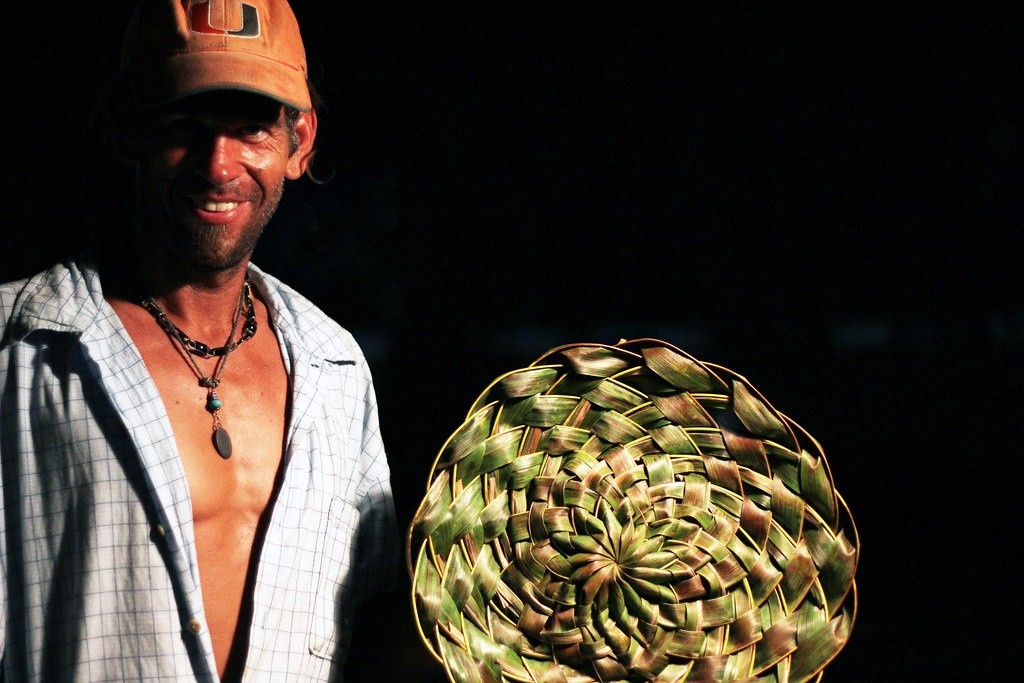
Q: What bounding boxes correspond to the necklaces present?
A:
[136,271,257,458]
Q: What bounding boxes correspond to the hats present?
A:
[119,0,313,113]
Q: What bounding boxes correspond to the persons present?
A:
[0,0,441,683]
